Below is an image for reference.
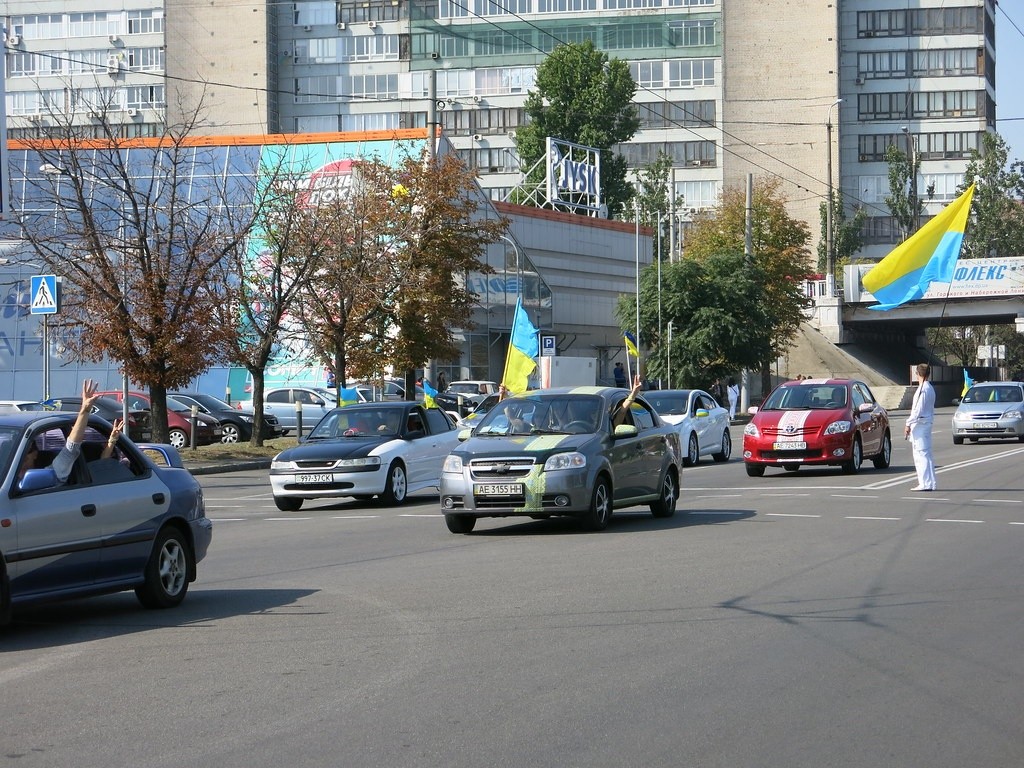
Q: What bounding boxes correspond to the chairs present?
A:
[408,415,423,431]
[81,441,104,462]
[789,389,809,407]
[0,440,77,486]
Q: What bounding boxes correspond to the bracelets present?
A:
[626,398,636,401]
[108,435,119,444]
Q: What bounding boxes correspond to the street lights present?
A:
[902,126,917,235]
[38,162,130,440]
[825,98,844,297]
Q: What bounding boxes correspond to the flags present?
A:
[861,181,975,312]
[961,368,973,398]
[423,379,440,410]
[624,331,644,358]
[499,298,540,400]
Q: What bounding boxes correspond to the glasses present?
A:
[532,414,547,419]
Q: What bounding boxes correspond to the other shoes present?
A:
[910,483,932,492]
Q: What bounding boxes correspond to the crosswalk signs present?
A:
[30,274,58,315]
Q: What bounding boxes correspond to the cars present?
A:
[442,380,498,413]
[0,410,216,635]
[324,374,474,418]
[455,390,537,434]
[742,377,892,478]
[0,392,285,448]
[952,381,1024,445]
[238,386,338,436]
[440,386,683,534]
[638,389,733,467]
[268,400,473,513]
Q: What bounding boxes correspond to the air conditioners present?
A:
[368,21,376,27]
[473,95,482,102]
[855,78,865,85]
[507,131,517,139]
[127,108,137,117]
[446,98,455,104]
[9,37,20,45]
[860,155,868,161]
[27,115,34,121]
[34,114,43,120]
[107,59,120,74]
[93,110,102,118]
[431,53,438,59]
[86,112,92,118]
[108,34,117,41]
[337,23,346,30]
[303,25,312,31]
[283,50,292,56]
[473,134,482,141]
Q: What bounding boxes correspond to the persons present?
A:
[327,365,336,388]
[824,385,846,408]
[437,371,448,394]
[498,383,565,434]
[415,376,423,389]
[795,374,813,381]
[974,391,985,402]
[904,363,938,492]
[1004,391,1020,401]
[666,398,687,416]
[710,378,725,409]
[613,362,627,388]
[85,418,126,461]
[727,375,740,421]
[377,410,409,436]
[563,374,644,435]
[2,377,101,496]
